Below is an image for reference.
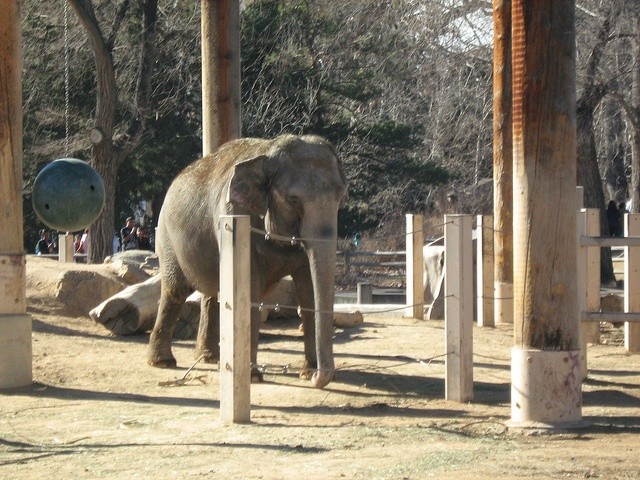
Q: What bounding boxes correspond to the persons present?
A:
[120,216,139,252]
[606,200,620,236]
[135,226,150,250]
[616,201,630,236]
[35,226,89,256]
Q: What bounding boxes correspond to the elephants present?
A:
[147,134,350,390]
